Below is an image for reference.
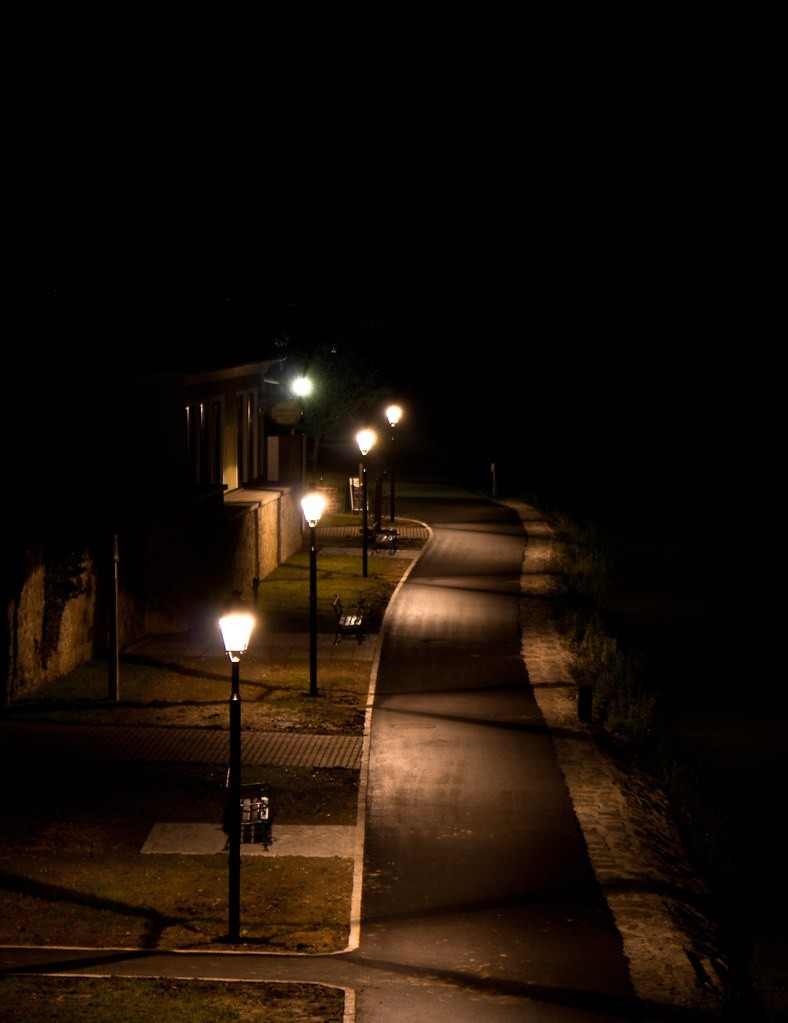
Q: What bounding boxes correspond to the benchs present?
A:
[223,765,273,845]
[327,593,364,645]
[372,521,397,557]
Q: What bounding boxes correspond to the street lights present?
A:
[355,427,378,582]
[288,373,318,538]
[299,490,331,698]
[384,405,404,523]
[216,592,258,943]
